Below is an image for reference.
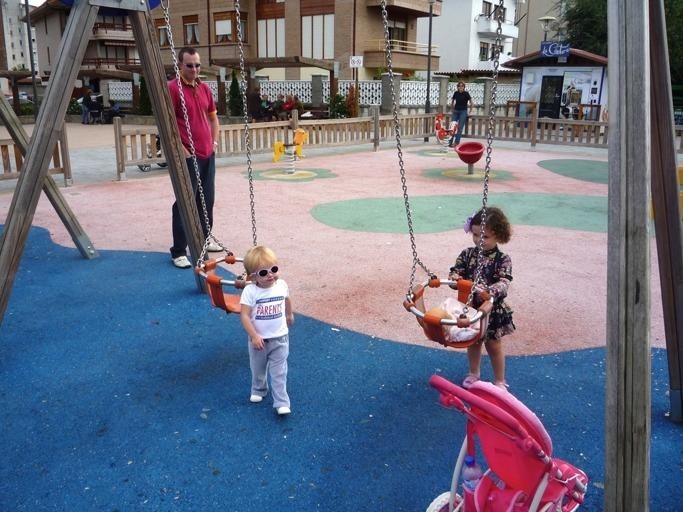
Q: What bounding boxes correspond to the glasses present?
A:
[247,266,279,279]
[182,63,201,68]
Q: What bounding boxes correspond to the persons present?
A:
[249,90,304,122]
[105,98,120,124]
[446,80,475,148]
[238,244,297,415]
[80,89,93,124]
[447,207,516,395]
[160,46,224,270]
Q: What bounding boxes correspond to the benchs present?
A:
[298,106,331,121]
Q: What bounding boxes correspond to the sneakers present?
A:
[277,406,291,414]
[249,394,263,403]
[205,243,223,251]
[171,257,192,268]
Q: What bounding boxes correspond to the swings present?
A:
[156,0,256,315]
[380,0,504,348]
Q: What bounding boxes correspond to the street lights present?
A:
[535,15,557,41]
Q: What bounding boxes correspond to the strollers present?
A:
[136,132,168,172]
[88,103,106,123]
[422,371,590,512]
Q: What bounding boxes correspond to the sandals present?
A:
[463,375,480,388]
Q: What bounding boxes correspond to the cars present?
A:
[3,90,35,105]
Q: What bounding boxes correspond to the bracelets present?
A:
[213,141,218,146]
